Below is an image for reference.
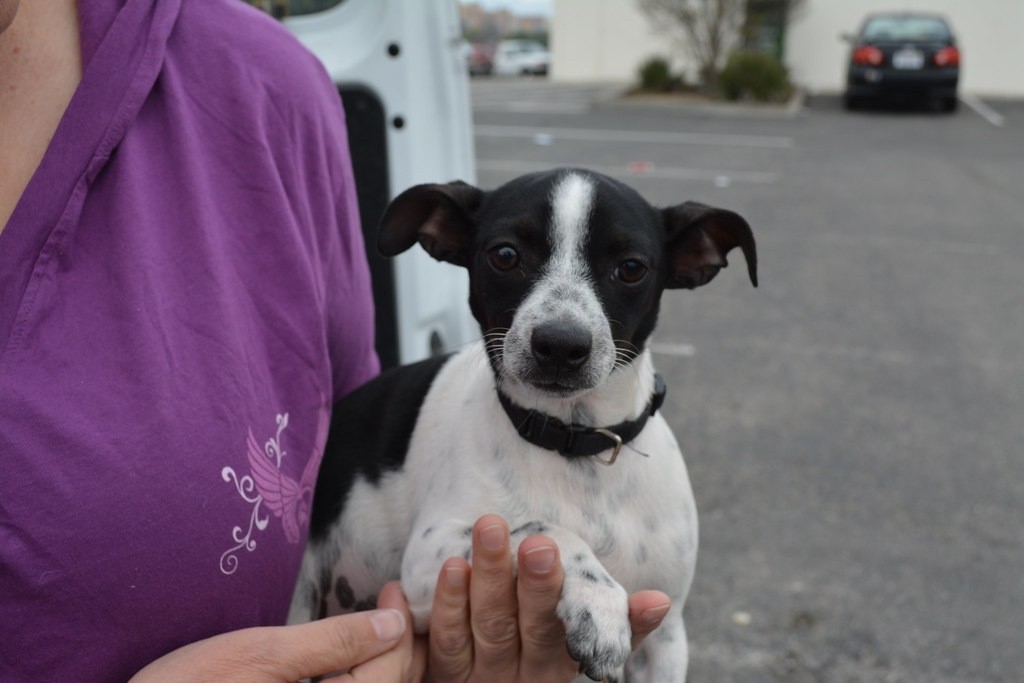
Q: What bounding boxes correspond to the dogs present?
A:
[283,166,758,683]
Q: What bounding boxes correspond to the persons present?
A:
[0,2,670,683]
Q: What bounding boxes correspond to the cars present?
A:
[493,39,547,77]
[841,11,959,112]
